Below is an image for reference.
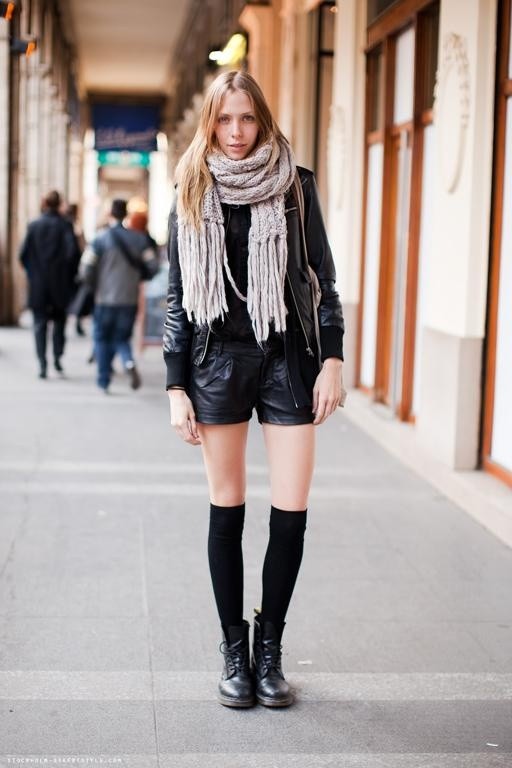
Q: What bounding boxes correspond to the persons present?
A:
[117,209,153,362]
[17,187,77,380]
[158,67,349,712]
[77,197,161,393]
[59,202,88,342]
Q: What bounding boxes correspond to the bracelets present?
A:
[165,386,188,392]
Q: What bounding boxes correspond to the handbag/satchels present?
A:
[304,260,349,409]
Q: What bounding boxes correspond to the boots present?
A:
[216,608,295,711]
